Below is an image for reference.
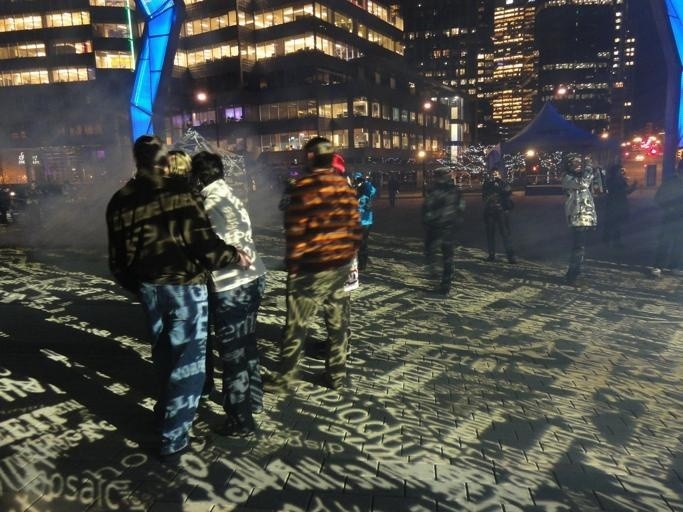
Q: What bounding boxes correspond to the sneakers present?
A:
[172,437,207,455]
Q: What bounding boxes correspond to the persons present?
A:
[353,171,377,270]
[263,137,360,394]
[387,175,398,208]
[23,181,45,234]
[559,152,598,286]
[422,170,466,294]
[606,153,638,233]
[650,161,683,276]
[194,151,263,435]
[481,167,517,266]
[164,151,192,178]
[105,135,251,459]
[0,183,10,225]
